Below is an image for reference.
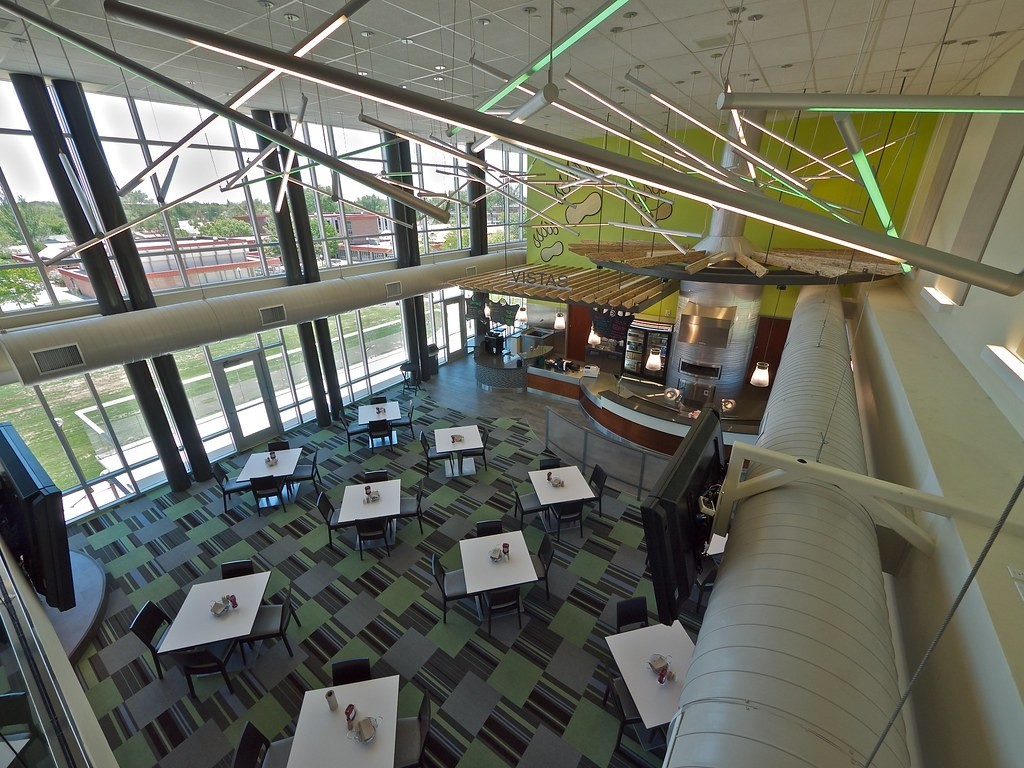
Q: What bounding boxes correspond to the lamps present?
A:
[554,303,565,329]
[485,303,491,318]
[751,284,789,387]
[517,297,527,321]
[646,278,668,370]
[588,265,603,345]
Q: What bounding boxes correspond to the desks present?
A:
[157,571,271,677]
[527,465,596,533]
[286,675,400,768]
[702,533,728,556]
[434,424,484,478]
[358,401,401,448]
[459,530,539,622]
[236,448,303,508]
[605,620,695,751]
[338,479,401,551]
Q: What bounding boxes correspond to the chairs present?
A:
[0,692,49,768]
[400,363,420,396]
[131,396,658,768]
[695,552,719,612]
